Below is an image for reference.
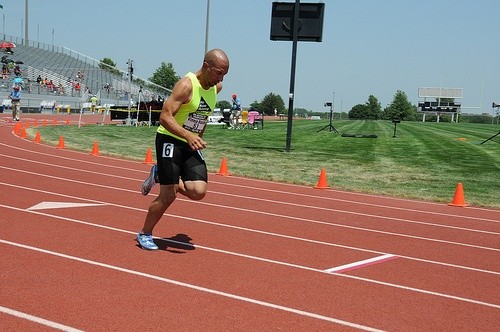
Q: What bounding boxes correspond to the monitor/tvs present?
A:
[270,2,325,41]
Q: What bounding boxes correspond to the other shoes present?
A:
[235,126,241,130]
[226,125,234,129]
[13,117,16,120]
[16,117,20,121]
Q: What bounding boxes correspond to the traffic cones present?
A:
[90,142,100,154]
[447,183,469,207]
[215,158,231,176]
[142,147,155,164]
[13,117,70,150]
[313,169,330,188]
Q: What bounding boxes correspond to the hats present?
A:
[232,94,236,99]
[13,85,19,91]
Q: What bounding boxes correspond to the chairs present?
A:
[233,111,264,130]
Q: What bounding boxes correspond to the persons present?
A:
[135,48,229,250]
[274,108,277,117]
[9,86,22,121]
[227,93,241,130]
[90,95,98,116]
[0,41,128,103]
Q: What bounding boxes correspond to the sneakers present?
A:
[141,164,158,196]
[136,232,159,250]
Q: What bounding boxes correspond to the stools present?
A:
[0,40,171,102]
